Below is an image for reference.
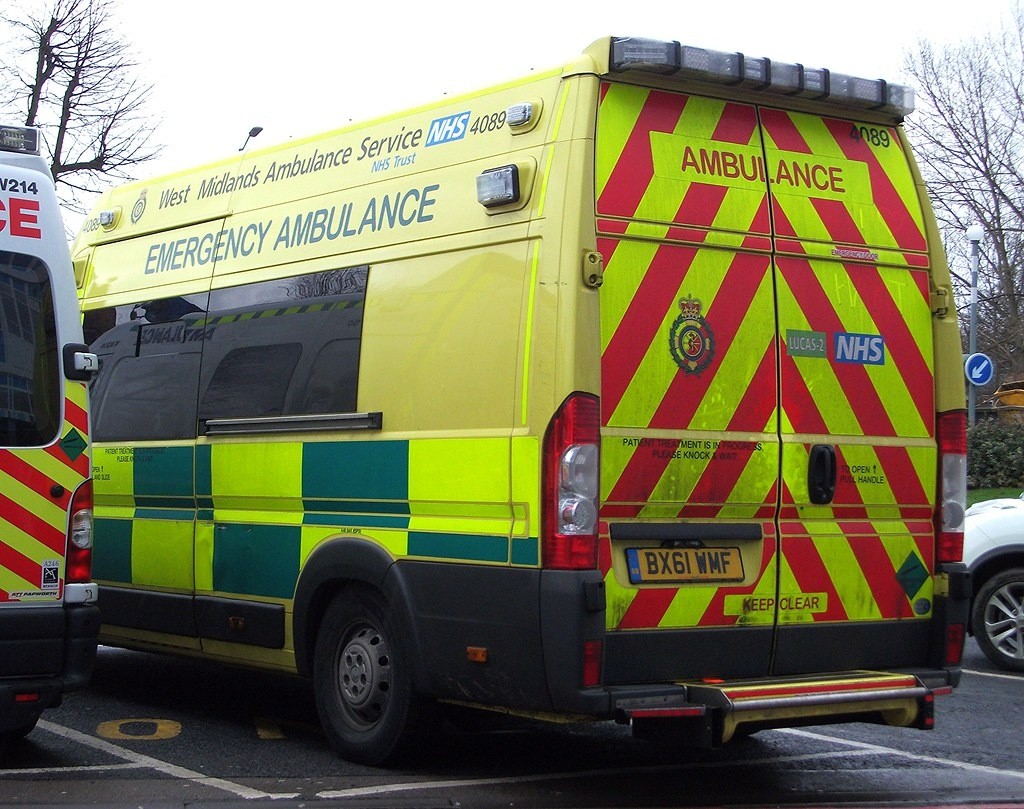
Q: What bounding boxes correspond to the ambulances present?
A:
[1,126,102,751]
[72,33,972,770]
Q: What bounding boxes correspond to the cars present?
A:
[959,490,1024,675]
